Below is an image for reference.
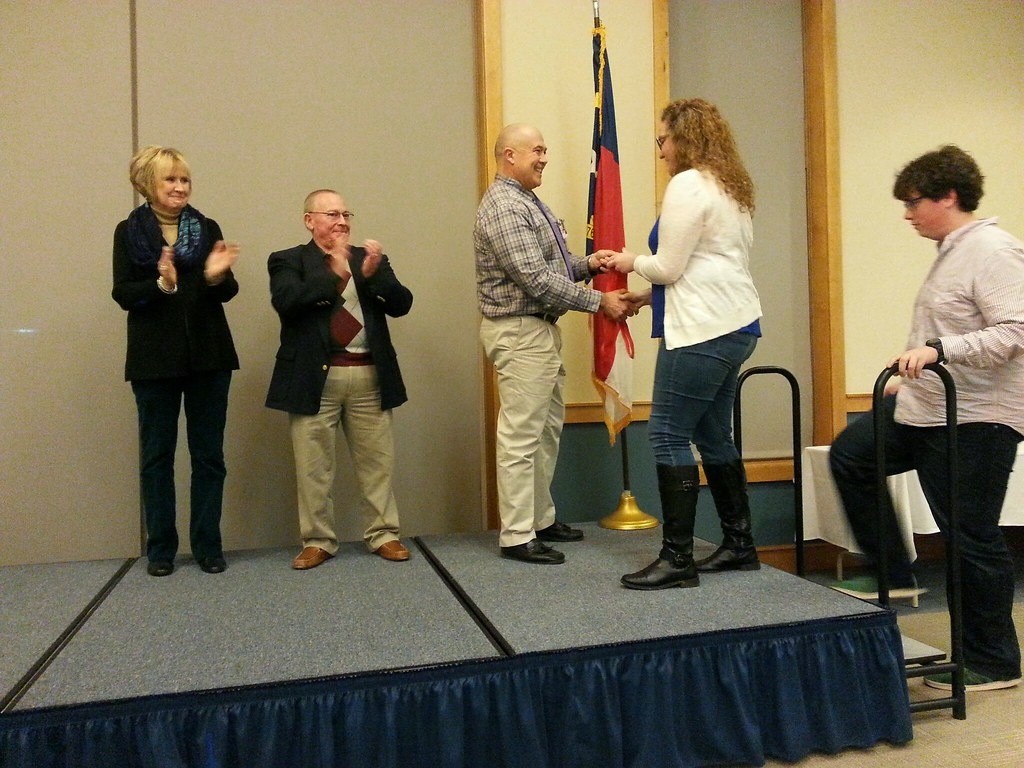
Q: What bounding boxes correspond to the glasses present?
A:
[904,195,925,210]
[310,210,354,219]
[655,134,670,150]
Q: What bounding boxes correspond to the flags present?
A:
[583,28,634,445]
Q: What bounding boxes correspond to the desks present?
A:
[794,442,1024,609]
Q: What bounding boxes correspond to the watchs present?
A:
[925,338,944,364]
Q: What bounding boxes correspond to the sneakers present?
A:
[829,573,918,597]
[924,662,1023,692]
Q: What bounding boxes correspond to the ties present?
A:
[532,196,576,284]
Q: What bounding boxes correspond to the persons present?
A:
[606,99,765,588]
[473,123,639,564]
[266,189,414,570]
[111,145,242,575]
[829,143,1024,690]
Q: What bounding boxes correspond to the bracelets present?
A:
[586,257,592,271]
[159,276,173,292]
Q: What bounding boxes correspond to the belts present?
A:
[529,310,559,325]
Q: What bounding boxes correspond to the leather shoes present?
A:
[199,556,226,572]
[293,547,330,569]
[376,540,411,562]
[501,538,565,564]
[147,560,174,576]
[535,517,584,542]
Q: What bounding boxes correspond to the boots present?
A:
[620,462,701,590]
[695,457,762,573]
